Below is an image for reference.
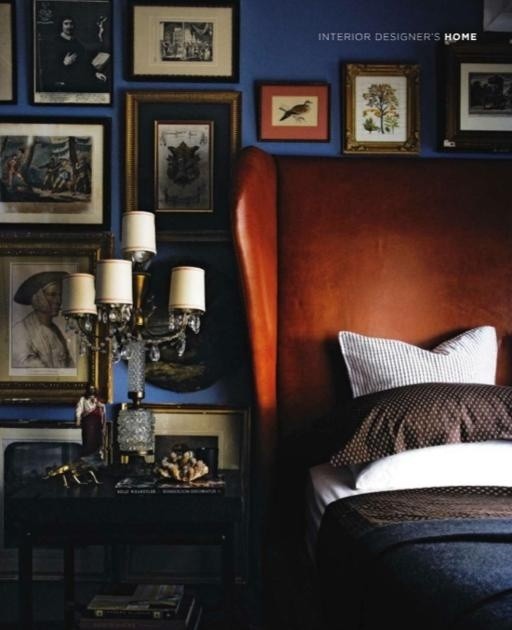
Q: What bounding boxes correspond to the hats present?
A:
[13,271,68,305]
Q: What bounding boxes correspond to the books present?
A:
[76,580,204,629]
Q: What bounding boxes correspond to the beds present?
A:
[232,145,512,629]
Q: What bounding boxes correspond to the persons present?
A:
[12,270,76,369]
[6,146,91,198]
[75,387,109,462]
[34,15,108,93]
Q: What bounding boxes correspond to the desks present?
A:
[3,469,242,629]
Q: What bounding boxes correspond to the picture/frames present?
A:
[123,91,242,246]
[0,116,112,232]
[1,231,114,407]
[0,419,107,582]
[123,405,256,586]
[255,2,512,155]
[31,1,112,106]
[1,1,17,103]
[127,3,240,85]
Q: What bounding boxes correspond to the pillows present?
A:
[350,442,511,490]
[336,326,500,399]
[331,383,512,469]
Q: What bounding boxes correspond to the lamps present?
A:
[61,212,208,466]
